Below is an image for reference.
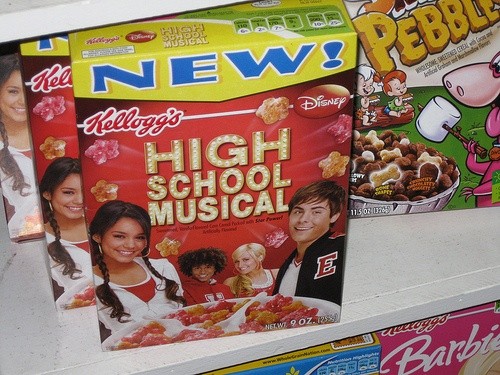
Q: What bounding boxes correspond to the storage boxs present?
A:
[0,0,500,375]
